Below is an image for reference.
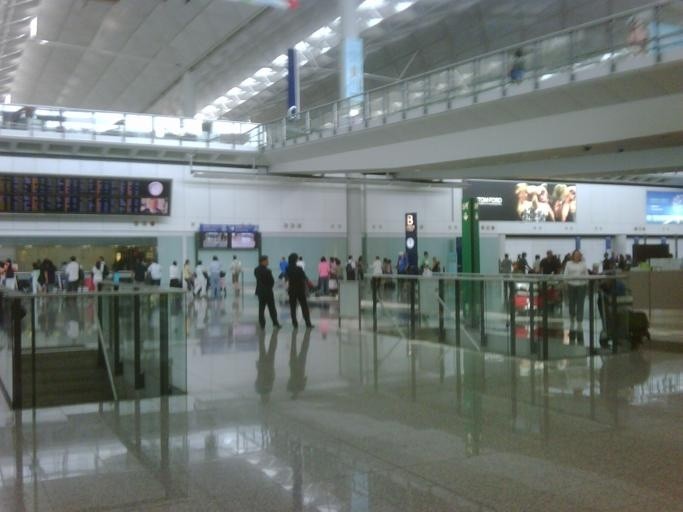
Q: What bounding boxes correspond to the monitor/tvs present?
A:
[200,231,255,249]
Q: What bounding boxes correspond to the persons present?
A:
[254,255,280,327]
[515,183,575,222]
[501,249,570,300]
[253,330,279,402]
[285,330,312,400]
[564,249,588,328]
[278,252,316,328]
[319,251,441,296]
[32,255,119,294]
[184,254,241,300]
[603,251,632,270]
[4,259,15,290]
[134,258,180,287]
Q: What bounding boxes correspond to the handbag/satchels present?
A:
[607,311,649,336]
[79,268,85,280]
[306,279,313,288]
[328,279,338,290]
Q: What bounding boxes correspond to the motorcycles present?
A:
[514,281,561,314]
[514,322,563,343]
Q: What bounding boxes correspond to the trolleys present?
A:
[595,288,650,349]
[595,268,634,310]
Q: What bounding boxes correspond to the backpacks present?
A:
[100,261,109,275]
